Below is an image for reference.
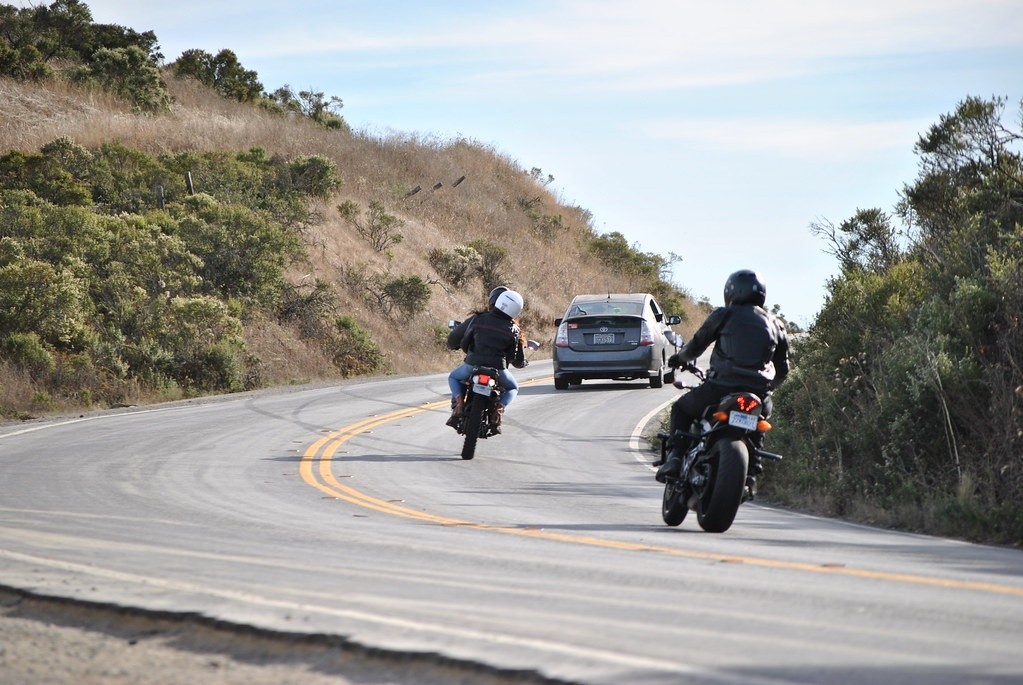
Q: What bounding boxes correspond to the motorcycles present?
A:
[447,319,542,461]
[653,329,785,533]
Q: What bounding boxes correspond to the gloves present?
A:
[667,354,681,368]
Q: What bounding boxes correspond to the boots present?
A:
[745,470,757,502]
[446,395,465,426]
[491,402,505,433]
[653,448,682,484]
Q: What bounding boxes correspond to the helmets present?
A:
[495,291,524,319]
[489,286,509,310]
[724,269,766,309]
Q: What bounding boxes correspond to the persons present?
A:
[448,290,524,424]
[445,286,529,435]
[654,271,792,502]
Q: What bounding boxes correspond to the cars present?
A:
[551,294,682,391]
